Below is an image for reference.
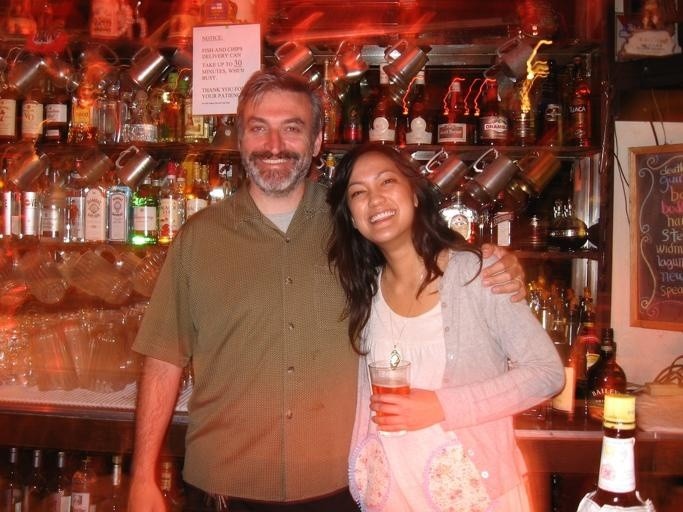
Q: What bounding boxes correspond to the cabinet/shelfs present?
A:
[0,0,615,383]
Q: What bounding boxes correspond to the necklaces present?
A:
[380,257,425,370]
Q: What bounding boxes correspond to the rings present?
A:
[516,276,524,285]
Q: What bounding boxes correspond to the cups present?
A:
[272,41,540,102]
[90,0,133,37]
[2,44,192,94]
[366,359,412,436]
[7,147,159,190]
[424,150,564,199]
[0,245,162,394]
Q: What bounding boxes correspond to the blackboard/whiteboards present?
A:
[628,144,683,331]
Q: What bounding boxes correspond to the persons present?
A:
[326,140,567,512]
[124,68,528,512]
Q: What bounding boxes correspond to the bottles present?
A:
[320,152,337,187]
[321,57,595,142]
[168,0,198,51]
[0,160,212,243]
[0,445,184,512]
[438,190,590,249]
[524,278,627,424]
[574,391,654,512]
[200,1,237,27]
[0,76,215,147]
